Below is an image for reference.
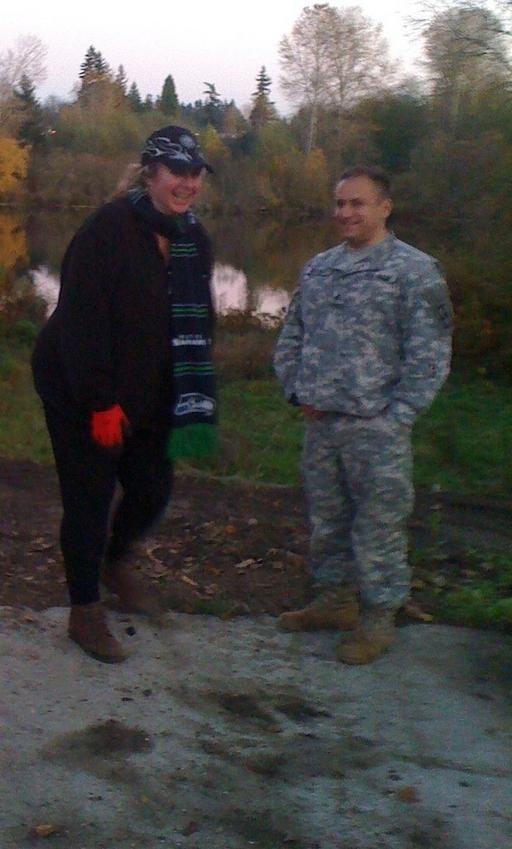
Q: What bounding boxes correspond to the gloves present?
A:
[90,403,131,447]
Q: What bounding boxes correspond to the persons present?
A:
[270,162,452,664]
[30,126,217,663]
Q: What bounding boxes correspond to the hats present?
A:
[139,124,215,175]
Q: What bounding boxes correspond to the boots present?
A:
[277,596,359,631]
[68,602,125,664]
[100,559,155,613]
[335,609,397,665]
